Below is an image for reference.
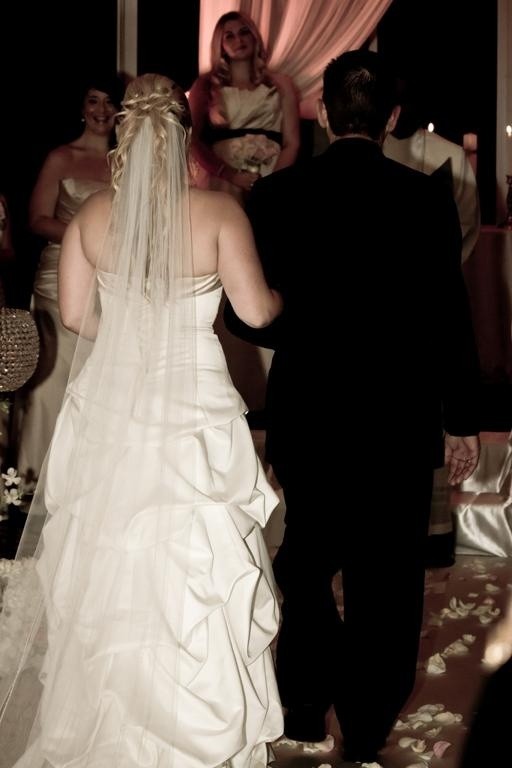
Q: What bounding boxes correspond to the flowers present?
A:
[230,131,278,194]
[0,468,25,507]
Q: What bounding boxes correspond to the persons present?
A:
[17,73,129,512]
[224,47,481,765]
[1,76,283,768]
[189,11,301,199]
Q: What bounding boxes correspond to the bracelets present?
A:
[216,160,227,177]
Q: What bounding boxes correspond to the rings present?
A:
[467,459,472,464]
